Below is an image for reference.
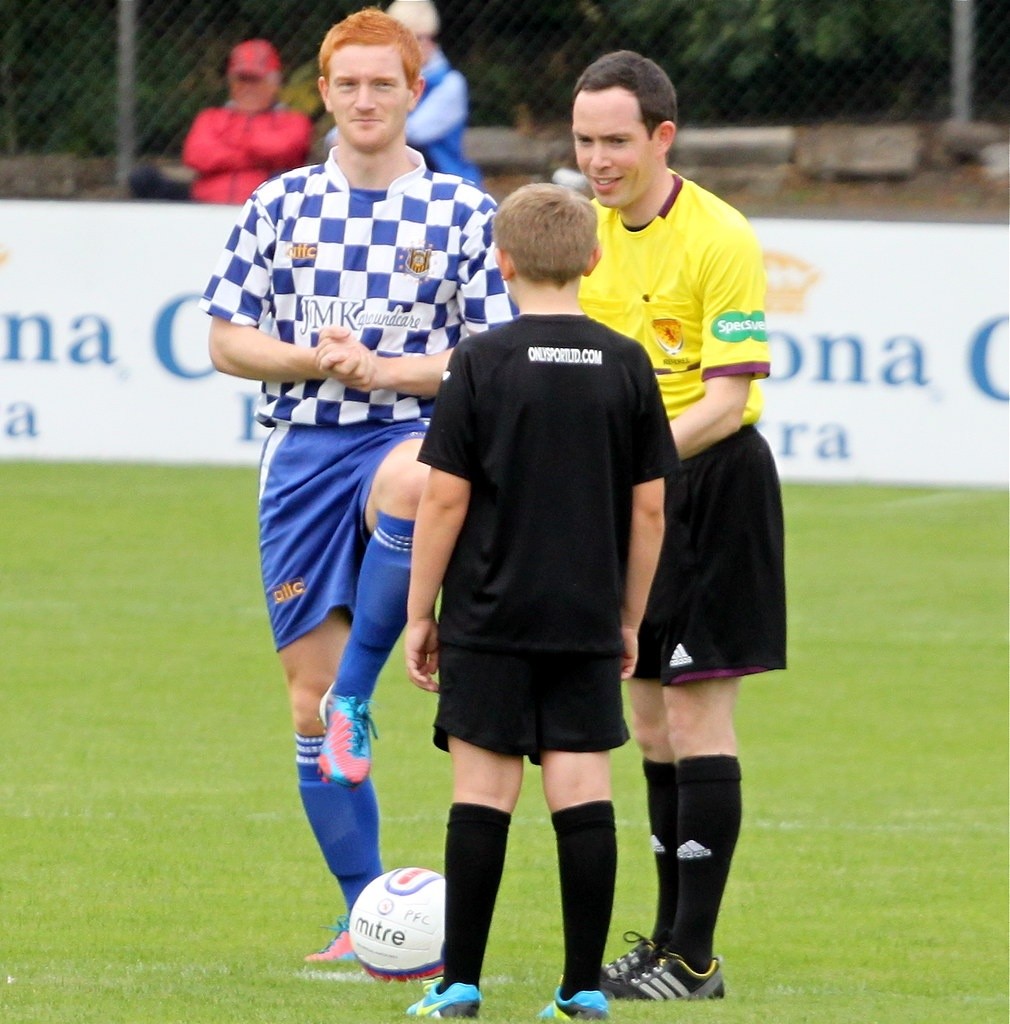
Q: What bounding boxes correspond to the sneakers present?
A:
[540,984,608,1023]
[601,956,724,1005]
[603,931,662,982]
[317,681,378,788]
[404,973,479,1021]
[302,918,357,961]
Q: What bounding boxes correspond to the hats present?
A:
[224,39,280,79]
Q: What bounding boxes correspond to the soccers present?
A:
[349,867,446,984]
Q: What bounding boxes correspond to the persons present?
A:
[406,183,683,1020]
[183,40,312,205]
[199,6,512,961]
[569,50,785,998]
[325,0,480,185]
